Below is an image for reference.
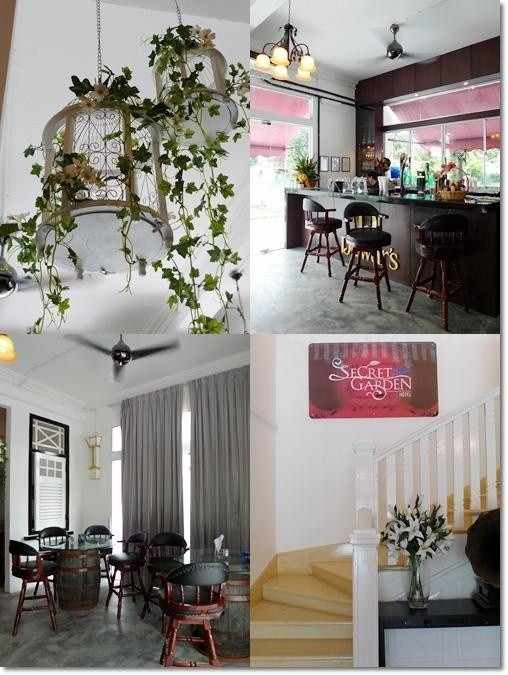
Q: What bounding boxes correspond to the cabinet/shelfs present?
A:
[379,626,500,667]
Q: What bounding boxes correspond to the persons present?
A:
[366,170,379,196]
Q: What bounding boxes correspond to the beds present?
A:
[174,549,250,659]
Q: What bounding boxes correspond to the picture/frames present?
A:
[320,156,350,172]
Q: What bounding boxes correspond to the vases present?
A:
[407,555,428,609]
[305,179,316,188]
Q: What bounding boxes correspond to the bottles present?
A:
[215,536,222,557]
[223,548,229,566]
[442,180,465,192]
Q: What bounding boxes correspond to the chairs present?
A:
[300,198,345,277]
[339,202,391,310]
[9,526,188,637]
[405,214,470,330]
[156,562,231,667]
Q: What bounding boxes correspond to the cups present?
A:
[327,176,389,197]
[240,552,250,569]
[78,534,85,546]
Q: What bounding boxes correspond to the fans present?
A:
[358,23,439,67]
[63,334,180,382]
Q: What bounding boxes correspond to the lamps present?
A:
[0,333,16,363]
[254,0,317,81]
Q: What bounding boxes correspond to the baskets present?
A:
[437,166,469,200]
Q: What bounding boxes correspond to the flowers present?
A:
[381,493,454,603]
[293,153,321,182]
